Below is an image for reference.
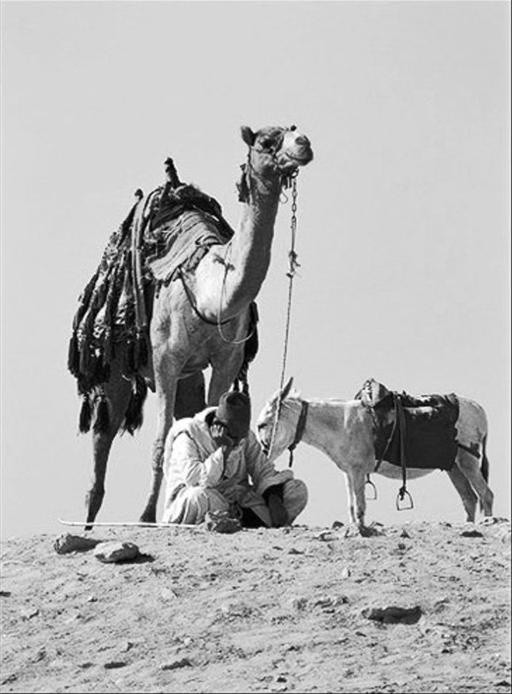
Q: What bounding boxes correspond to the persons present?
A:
[163,393,307,528]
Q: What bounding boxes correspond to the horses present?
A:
[256,375,494,526]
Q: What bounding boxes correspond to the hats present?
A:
[215,391,251,439]
